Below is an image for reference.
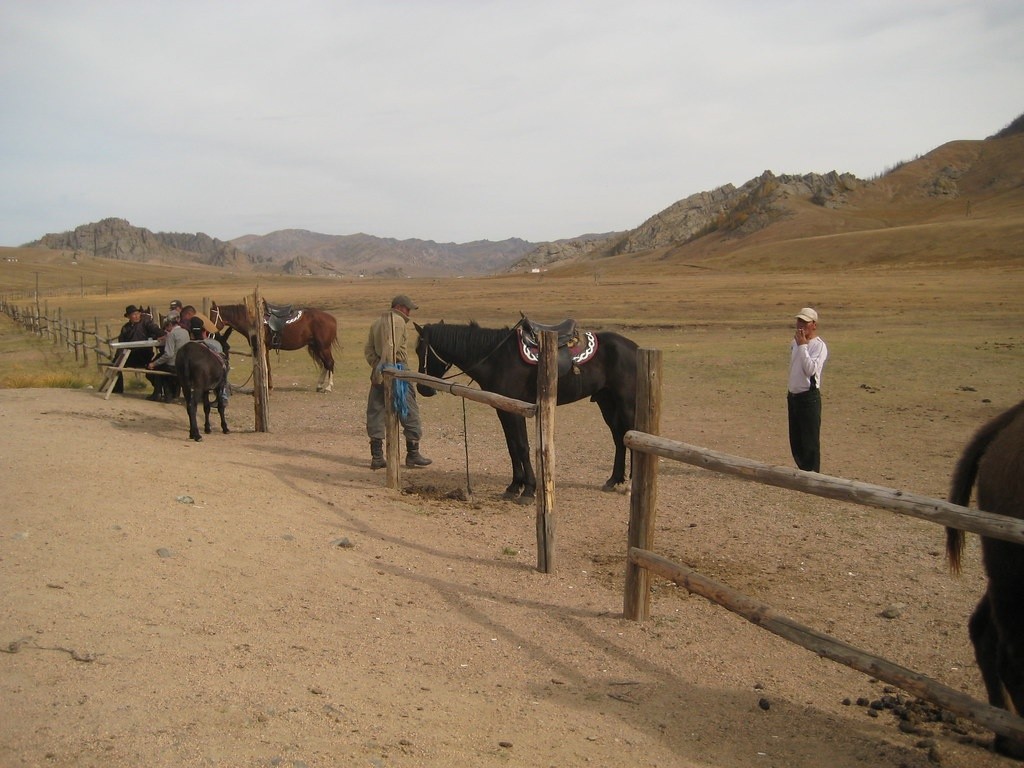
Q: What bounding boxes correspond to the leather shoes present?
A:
[406,451,432,466]
[370,450,386,468]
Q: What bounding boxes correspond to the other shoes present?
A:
[106,385,123,392]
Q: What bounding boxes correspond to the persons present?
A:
[365,295,433,470]
[787,308,827,473]
[106,300,231,407]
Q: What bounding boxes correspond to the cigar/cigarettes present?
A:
[798,329,804,330]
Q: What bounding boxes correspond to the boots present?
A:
[164,385,173,402]
[146,384,162,401]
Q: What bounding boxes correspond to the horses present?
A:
[945,400,1024,759]
[412,320,641,506]
[174,324,234,442]
[210,300,337,395]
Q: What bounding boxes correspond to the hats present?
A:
[168,300,182,309]
[124,305,141,317]
[391,295,419,309]
[162,317,173,328]
[794,308,819,323]
[167,310,180,319]
